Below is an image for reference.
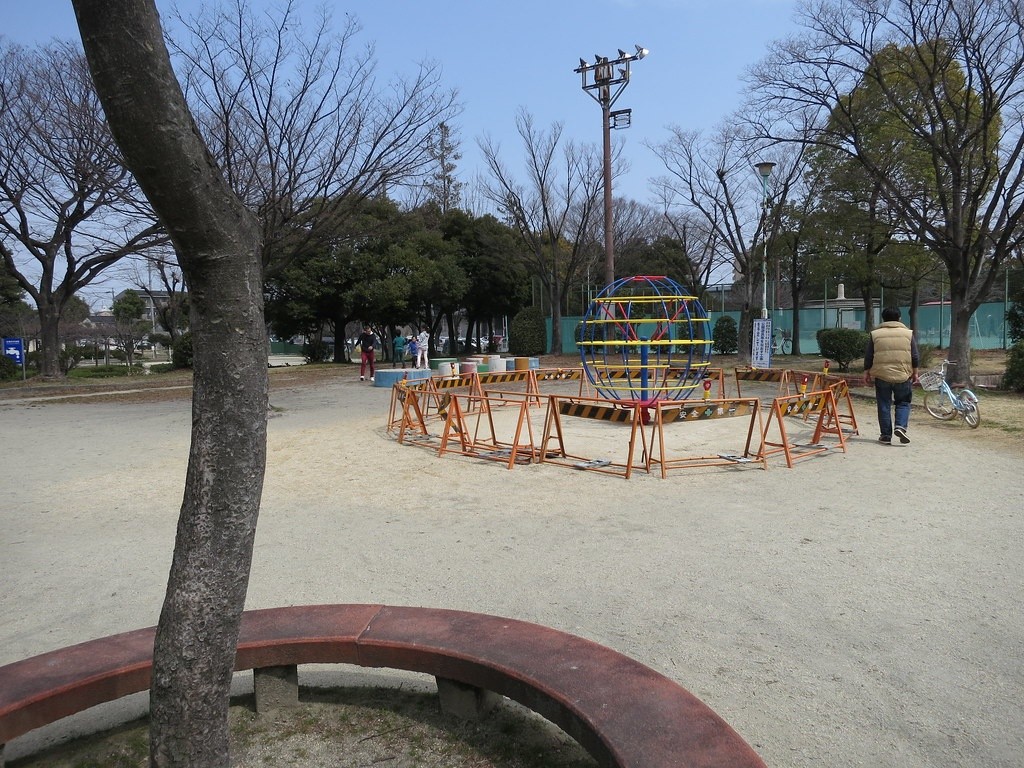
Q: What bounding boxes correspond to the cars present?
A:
[323,335,507,353]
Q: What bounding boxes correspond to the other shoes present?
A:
[893,428,911,443]
[879,434,891,445]
[371,377,375,382]
[360,375,365,381]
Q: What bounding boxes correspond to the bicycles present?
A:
[918,359,982,428]
[768,324,793,357]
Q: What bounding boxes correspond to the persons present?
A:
[355,325,376,382]
[864,306,918,445]
[408,324,431,369]
[987,314,1000,338]
[392,329,406,369]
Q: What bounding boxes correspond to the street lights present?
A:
[751,161,778,366]
[574,42,649,356]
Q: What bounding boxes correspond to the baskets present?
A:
[781,329,791,337]
[917,369,943,391]
